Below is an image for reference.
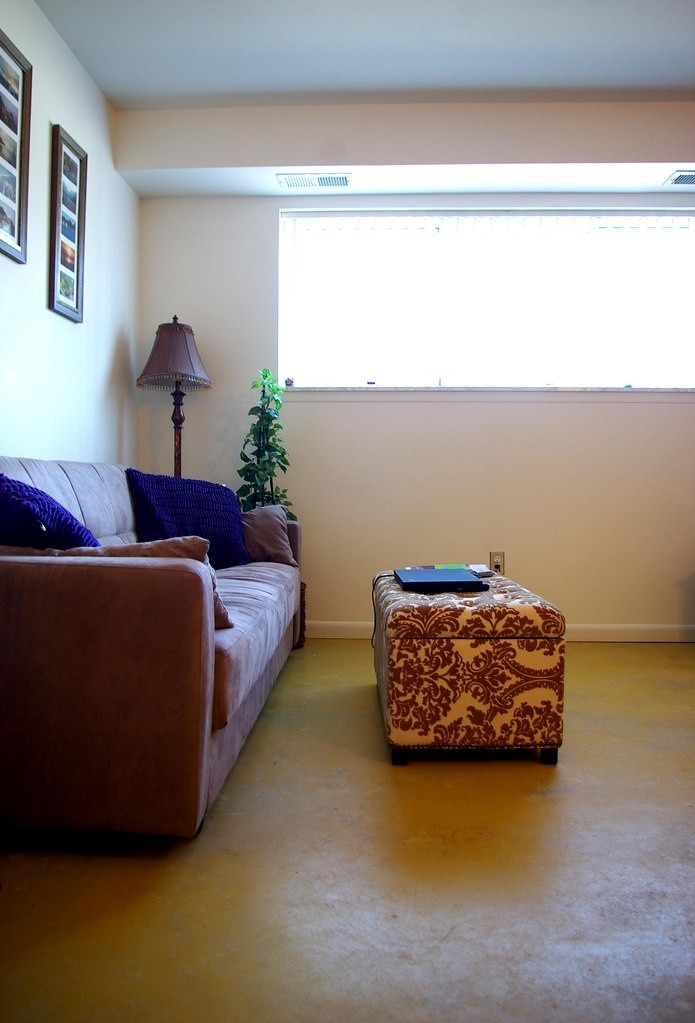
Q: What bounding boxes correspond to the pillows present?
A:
[125,468,250,570]
[0,535,234,630]
[241,505,300,567]
[0,473,101,551]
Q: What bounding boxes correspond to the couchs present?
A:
[0,457,308,847]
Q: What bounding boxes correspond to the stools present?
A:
[372,564,565,765]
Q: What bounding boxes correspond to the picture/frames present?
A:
[0,29,34,264]
[49,124,89,323]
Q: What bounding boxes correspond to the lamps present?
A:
[136,314,213,479]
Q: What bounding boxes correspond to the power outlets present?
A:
[489,552,504,575]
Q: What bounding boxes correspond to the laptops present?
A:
[393,569,490,594]
